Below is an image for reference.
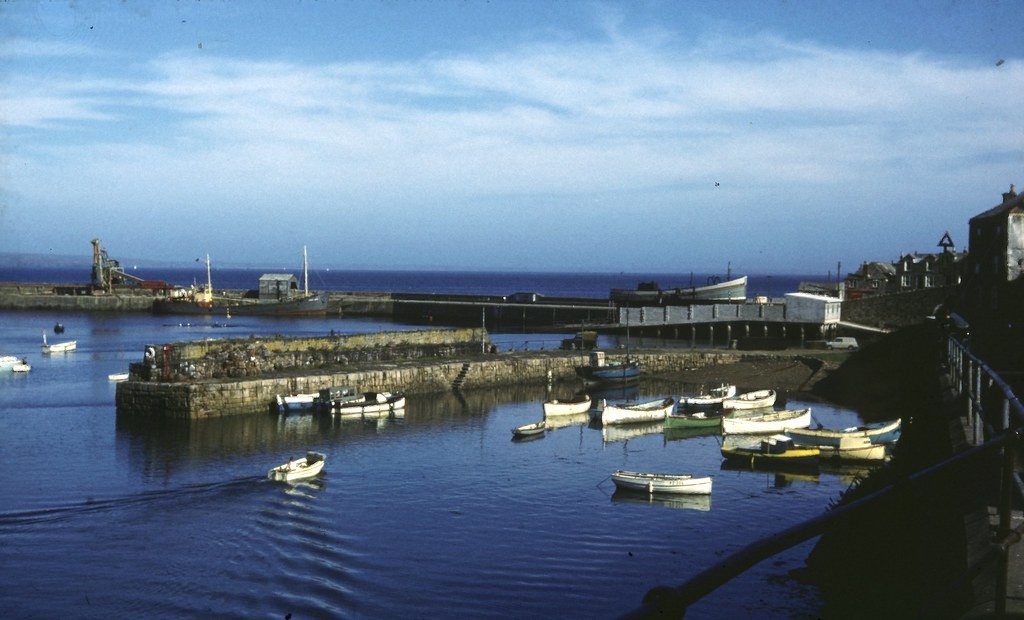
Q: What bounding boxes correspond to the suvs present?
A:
[827,337,860,350]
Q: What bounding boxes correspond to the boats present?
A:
[783,417,903,460]
[575,309,641,384]
[510,421,546,435]
[108,371,130,381]
[277,409,319,433]
[313,385,406,414]
[312,409,405,430]
[611,487,713,513]
[676,383,737,413]
[722,408,812,435]
[542,390,592,416]
[664,426,724,442]
[41,329,78,353]
[602,419,665,449]
[575,381,641,401]
[721,434,821,466]
[820,457,895,485]
[267,450,327,481]
[543,411,590,430]
[276,391,320,411]
[152,245,330,317]
[611,469,715,496]
[722,389,776,409]
[600,397,676,426]
[511,431,545,444]
[721,459,821,489]
[0,356,32,373]
[664,408,734,429]
[609,261,749,302]
